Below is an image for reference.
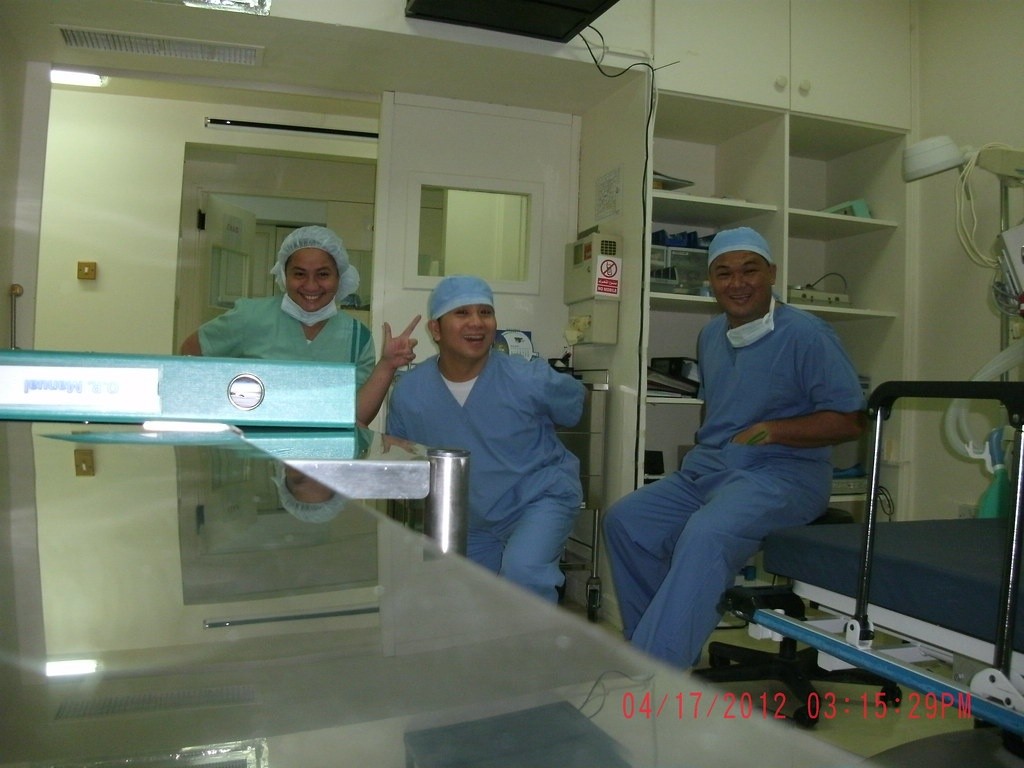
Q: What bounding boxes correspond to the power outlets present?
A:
[958,504,979,519]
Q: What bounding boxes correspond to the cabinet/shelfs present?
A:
[652,0,916,133]
[567,83,923,631]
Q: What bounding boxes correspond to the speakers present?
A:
[404,0,619,43]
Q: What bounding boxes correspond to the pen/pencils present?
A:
[559,345,571,363]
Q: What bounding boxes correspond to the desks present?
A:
[0,419,872,768]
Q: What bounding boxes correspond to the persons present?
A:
[384,275,587,609]
[236,430,417,524]
[601,226,868,674]
[181,225,422,427]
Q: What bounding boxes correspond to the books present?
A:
[646,366,700,398]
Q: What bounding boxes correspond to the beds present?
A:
[757,379,1024,768]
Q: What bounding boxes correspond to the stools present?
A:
[693,508,903,736]
